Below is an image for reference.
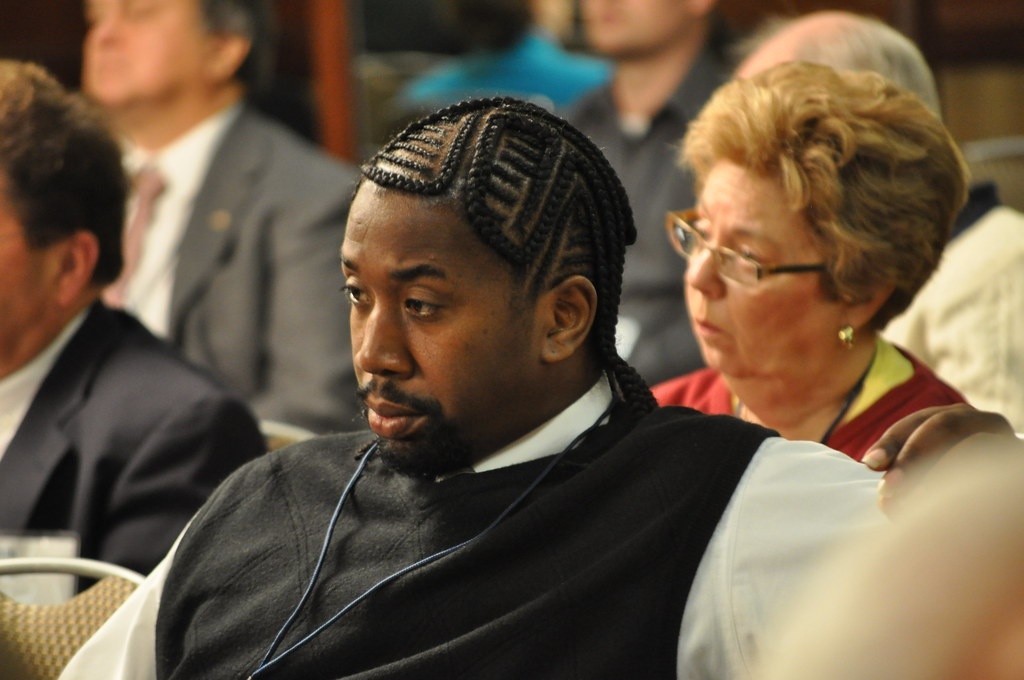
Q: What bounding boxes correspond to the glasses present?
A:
[664,207,825,286]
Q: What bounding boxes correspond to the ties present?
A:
[102,166,166,312]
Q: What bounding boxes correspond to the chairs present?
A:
[0,558,149,680]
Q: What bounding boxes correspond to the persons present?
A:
[400,0,749,390]
[52,94,1016,680]
[0,59,269,611]
[645,61,975,464]
[75,0,371,441]
[733,12,1024,432]
